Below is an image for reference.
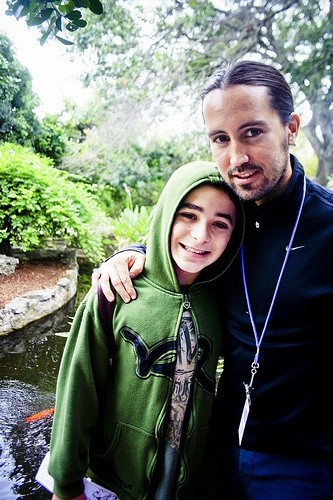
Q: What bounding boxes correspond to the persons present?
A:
[89,61,332,500]
[48,160,246,500]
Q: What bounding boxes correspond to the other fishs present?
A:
[25,407,54,424]
